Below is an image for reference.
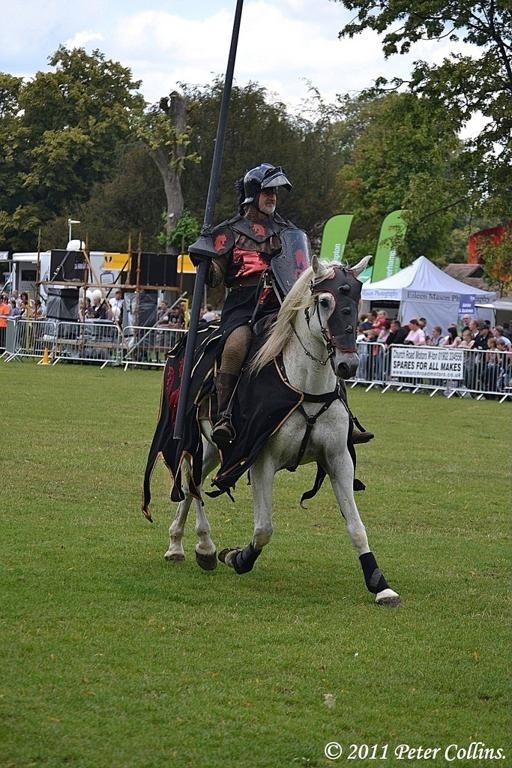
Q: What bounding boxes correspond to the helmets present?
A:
[237,162,296,210]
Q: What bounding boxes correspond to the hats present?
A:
[476,321,491,330]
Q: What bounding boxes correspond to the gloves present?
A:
[188,252,214,268]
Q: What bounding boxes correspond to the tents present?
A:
[357,255,498,337]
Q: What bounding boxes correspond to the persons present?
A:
[190,163,374,448]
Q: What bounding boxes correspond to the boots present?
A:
[338,379,375,446]
[210,369,239,451]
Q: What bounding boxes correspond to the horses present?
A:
[162,255,400,606]
